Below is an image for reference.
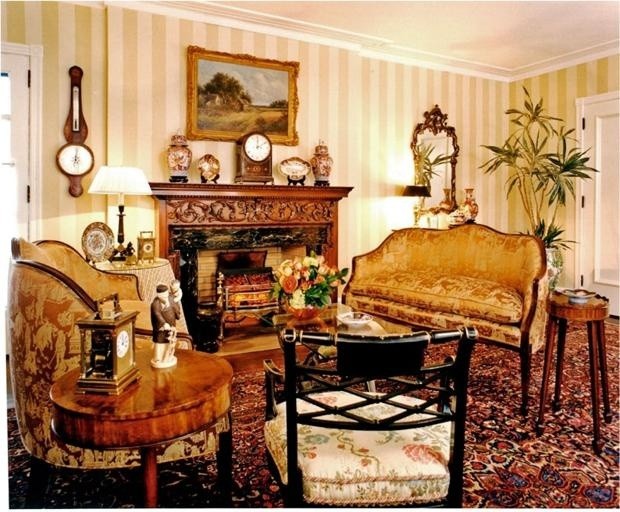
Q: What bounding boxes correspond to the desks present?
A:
[91,258,197,350]
[534,287,615,458]
[38,336,237,509]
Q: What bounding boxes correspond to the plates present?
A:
[335,311,373,327]
[278,156,312,179]
[197,154,221,179]
[81,222,115,263]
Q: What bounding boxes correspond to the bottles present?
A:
[440,188,452,208]
[310,145,334,182]
[124,242,137,264]
[465,188,479,221]
[166,135,193,177]
[194,300,222,353]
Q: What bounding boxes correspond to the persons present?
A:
[148,284,174,363]
[165,281,182,361]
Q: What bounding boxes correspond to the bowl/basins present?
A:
[561,288,599,304]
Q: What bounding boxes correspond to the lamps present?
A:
[399,185,433,200]
[87,163,156,263]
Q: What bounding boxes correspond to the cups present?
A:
[447,211,465,225]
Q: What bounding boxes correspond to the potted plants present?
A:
[476,82,604,290]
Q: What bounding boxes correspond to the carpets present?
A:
[6,342,620,509]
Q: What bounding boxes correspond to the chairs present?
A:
[249,325,481,509]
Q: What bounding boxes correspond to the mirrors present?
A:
[409,103,462,224]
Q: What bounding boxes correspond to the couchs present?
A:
[338,221,557,418]
[6,237,237,509]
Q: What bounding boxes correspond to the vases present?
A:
[438,188,455,211]
[308,137,335,181]
[166,127,193,178]
[283,286,327,320]
[461,187,479,222]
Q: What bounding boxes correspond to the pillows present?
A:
[9,234,59,271]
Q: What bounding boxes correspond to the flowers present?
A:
[264,250,350,308]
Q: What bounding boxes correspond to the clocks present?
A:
[233,130,275,185]
[73,291,144,398]
[53,64,100,200]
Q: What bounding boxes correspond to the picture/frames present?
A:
[183,42,302,149]
